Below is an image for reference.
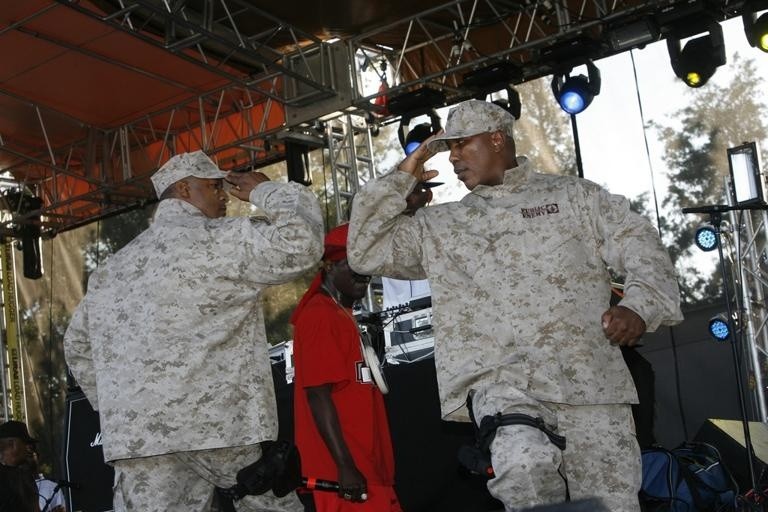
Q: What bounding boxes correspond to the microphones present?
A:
[49,478,80,490]
[299,477,340,492]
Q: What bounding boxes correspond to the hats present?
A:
[150,149,230,200]
[0,420,41,444]
[427,100,515,153]
[324,224,349,261]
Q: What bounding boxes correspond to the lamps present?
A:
[694,224,738,341]
[659,0,767,89]
[385,34,601,154]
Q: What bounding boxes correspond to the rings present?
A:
[344,492,352,500]
[360,492,368,501]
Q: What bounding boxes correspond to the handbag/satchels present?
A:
[639,438,740,511]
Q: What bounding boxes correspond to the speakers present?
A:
[64,390,114,512]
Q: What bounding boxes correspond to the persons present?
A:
[60,146,328,512]
[0,420,40,512]
[344,99,686,512]
[19,450,67,512]
[289,223,402,512]
